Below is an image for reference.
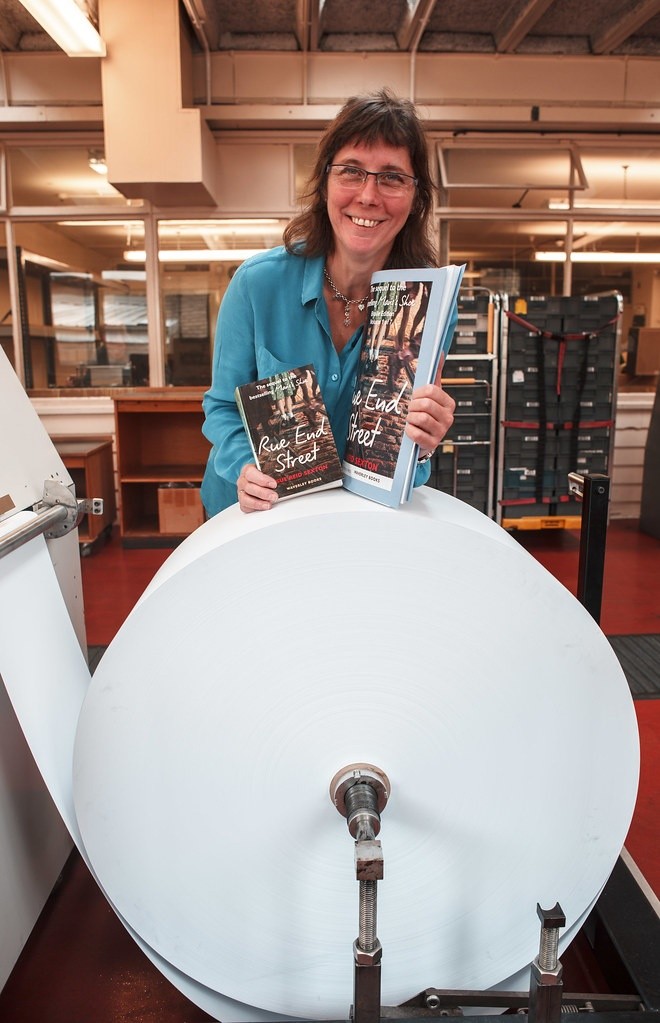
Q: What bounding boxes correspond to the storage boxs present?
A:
[157,487,206,533]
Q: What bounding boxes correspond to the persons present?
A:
[201,94,455,519]
[363,282,432,379]
[243,366,320,454]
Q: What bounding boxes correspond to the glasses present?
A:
[326,163,419,198]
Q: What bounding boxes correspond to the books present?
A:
[341,265,465,508]
[235,363,346,503]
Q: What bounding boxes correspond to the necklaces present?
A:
[323,268,369,327]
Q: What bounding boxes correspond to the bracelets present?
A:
[418,449,435,464]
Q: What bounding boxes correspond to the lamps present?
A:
[19,0,108,58]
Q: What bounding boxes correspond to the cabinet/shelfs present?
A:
[50,436,118,556]
[608,384,656,519]
[110,393,215,541]
